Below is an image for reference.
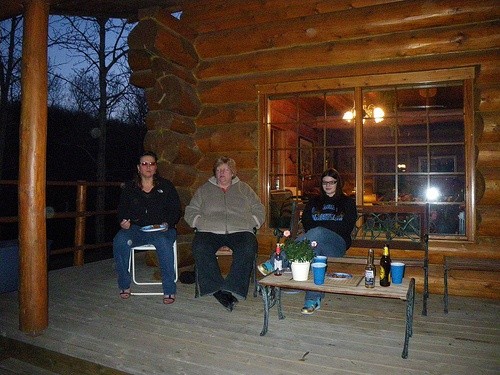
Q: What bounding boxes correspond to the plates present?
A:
[140,224,166,232]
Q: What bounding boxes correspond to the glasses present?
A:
[322,181,338,185]
[142,161,156,166]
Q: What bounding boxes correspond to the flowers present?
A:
[278,230,317,263]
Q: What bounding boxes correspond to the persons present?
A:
[113,151,181,305]
[256,169,356,313]
[183,156,266,312]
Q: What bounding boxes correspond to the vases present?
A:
[290,261,311,281]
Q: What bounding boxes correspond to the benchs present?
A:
[443,255,500,314]
[274,200,430,316]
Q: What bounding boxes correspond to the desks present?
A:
[271,186,303,218]
[258,271,415,359]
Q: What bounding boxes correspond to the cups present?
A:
[314,255,328,269]
[311,262,328,285]
[390,263,406,284]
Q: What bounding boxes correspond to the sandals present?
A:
[163,295,175,304]
[120,286,131,299]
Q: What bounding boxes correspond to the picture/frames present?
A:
[298,135,314,179]
[417,154,458,179]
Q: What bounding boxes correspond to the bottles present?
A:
[364,248,376,288]
[378,242,391,287]
[274,242,283,276]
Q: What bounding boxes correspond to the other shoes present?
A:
[256,259,273,276]
[300,303,321,314]
[213,290,239,312]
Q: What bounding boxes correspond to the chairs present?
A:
[195,245,257,298]
[128,239,178,296]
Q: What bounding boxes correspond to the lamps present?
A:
[343,98,386,124]
